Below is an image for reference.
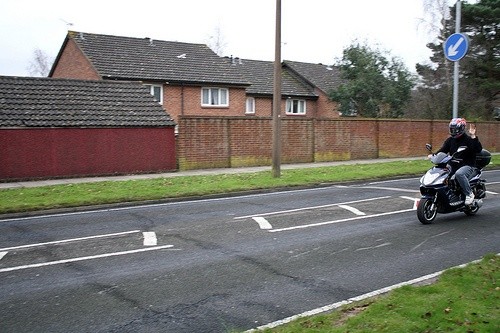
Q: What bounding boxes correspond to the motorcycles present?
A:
[416,143,493,225]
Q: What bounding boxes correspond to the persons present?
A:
[427,118,483,205]
